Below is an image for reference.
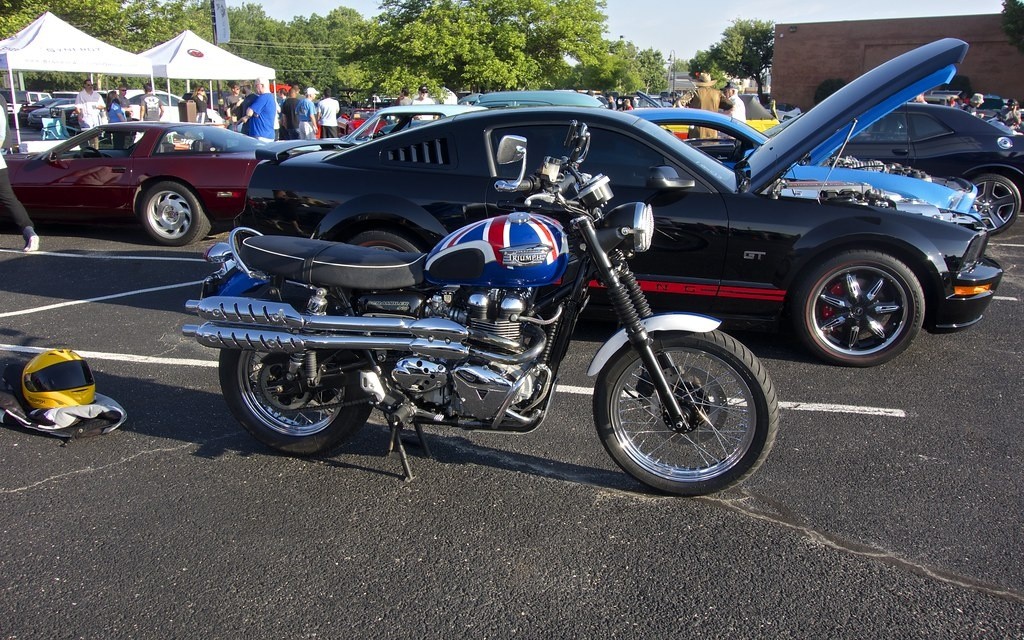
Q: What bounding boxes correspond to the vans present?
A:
[0,90,52,123]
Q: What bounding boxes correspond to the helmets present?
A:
[22,349,96,409]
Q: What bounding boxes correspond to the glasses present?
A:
[86,84,93,86]
[199,90,205,92]
[421,91,428,93]
[121,90,127,91]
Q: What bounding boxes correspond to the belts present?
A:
[301,120,311,122]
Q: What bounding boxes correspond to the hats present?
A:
[691,73,719,86]
[418,85,430,91]
[720,82,739,89]
[1005,99,1018,105]
[306,88,319,94]
[970,93,985,104]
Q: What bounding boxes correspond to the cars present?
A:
[234,37,1002,368]
[764,102,802,123]
[275,105,494,150]
[18,96,78,129]
[336,108,387,138]
[629,89,670,109]
[124,92,226,129]
[182,90,231,115]
[825,102,1024,236]
[458,90,606,111]
[0,121,266,247]
[621,64,979,226]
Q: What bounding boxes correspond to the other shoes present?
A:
[22,231,39,252]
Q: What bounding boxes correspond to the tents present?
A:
[137,29,277,109]
[0,9,155,149]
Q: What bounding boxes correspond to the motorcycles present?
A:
[182,116,780,498]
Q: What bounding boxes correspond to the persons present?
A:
[365,85,439,124]
[106,85,134,150]
[667,72,747,147]
[139,83,165,133]
[912,92,1024,136]
[75,78,109,150]
[586,88,633,111]
[0,93,41,253]
[224,76,340,144]
[191,85,209,124]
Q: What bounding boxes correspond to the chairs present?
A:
[41,117,79,142]
